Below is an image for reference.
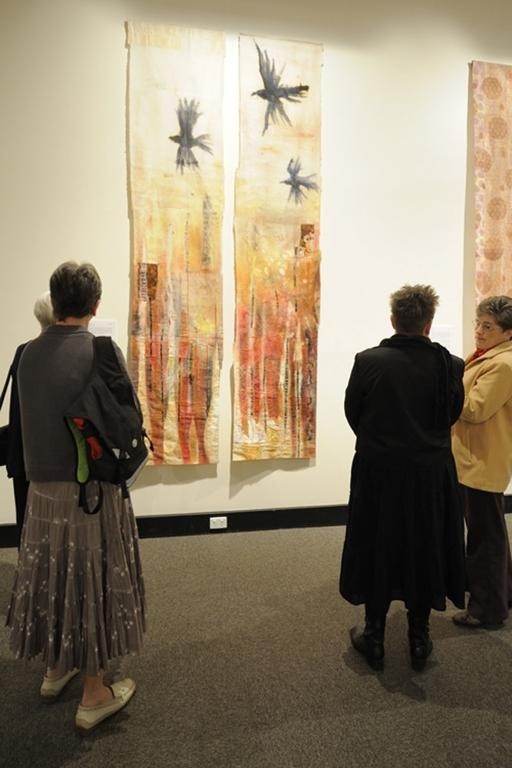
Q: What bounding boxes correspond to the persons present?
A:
[334,284,475,672]
[444,295,509,633]
[3,292,61,572]
[3,257,156,734]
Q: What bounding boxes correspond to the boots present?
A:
[350,614,385,660]
[407,611,433,671]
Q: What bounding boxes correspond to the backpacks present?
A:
[64,336,148,514]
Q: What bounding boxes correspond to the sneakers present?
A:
[452,611,505,631]
[39,666,81,704]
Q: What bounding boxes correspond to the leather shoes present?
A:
[75,678,136,737]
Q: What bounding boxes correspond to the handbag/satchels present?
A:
[0,424,10,466]
